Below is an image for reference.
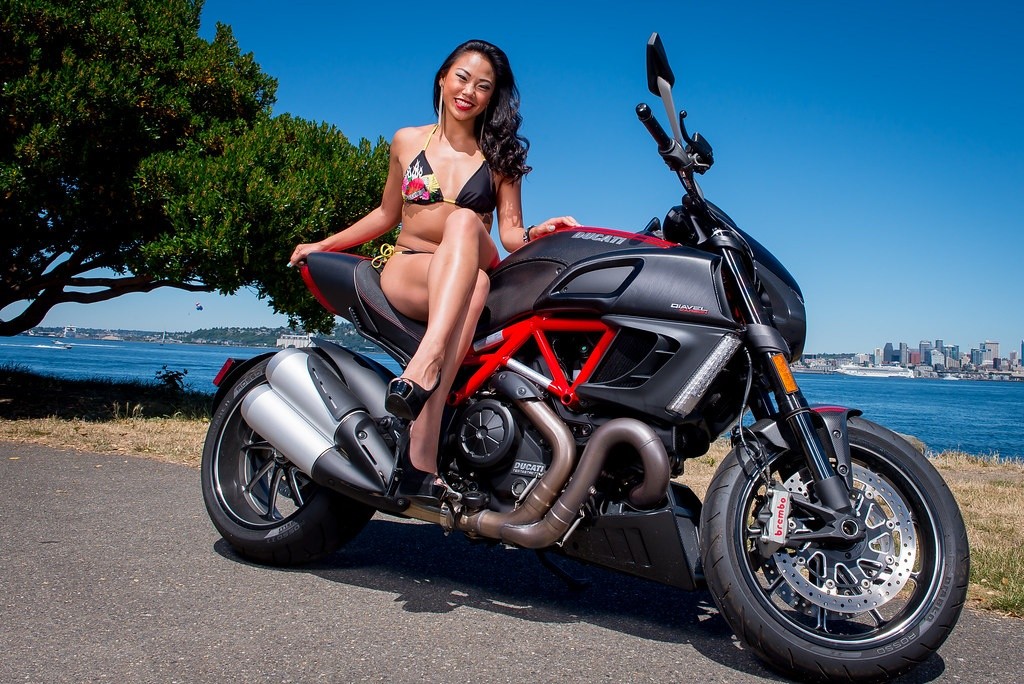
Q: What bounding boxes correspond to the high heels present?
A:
[385,370,440,430]
[386,428,448,505]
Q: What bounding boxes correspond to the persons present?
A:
[287,40,583,502]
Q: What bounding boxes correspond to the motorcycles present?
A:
[197,29,970,684]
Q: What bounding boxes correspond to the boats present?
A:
[942,376,959,380]
[51,339,63,345]
[59,345,73,349]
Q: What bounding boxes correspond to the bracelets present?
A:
[523,225,536,243]
